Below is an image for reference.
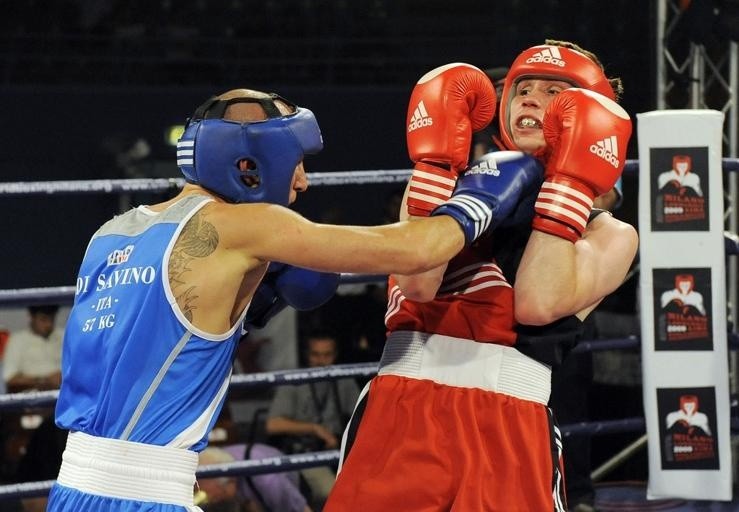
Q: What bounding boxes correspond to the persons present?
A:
[199,193,405,509]
[104,136,182,210]
[325,39,638,512]
[1,304,67,482]
[46,89,544,511]
[471,68,623,210]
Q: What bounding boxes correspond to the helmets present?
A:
[674,273,697,295]
[679,396,699,414]
[176,94,326,208]
[672,155,693,175]
[498,41,619,164]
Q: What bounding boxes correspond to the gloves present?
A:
[403,61,498,217]
[532,87,634,244]
[244,261,342,331]
[431,150,545,249]
